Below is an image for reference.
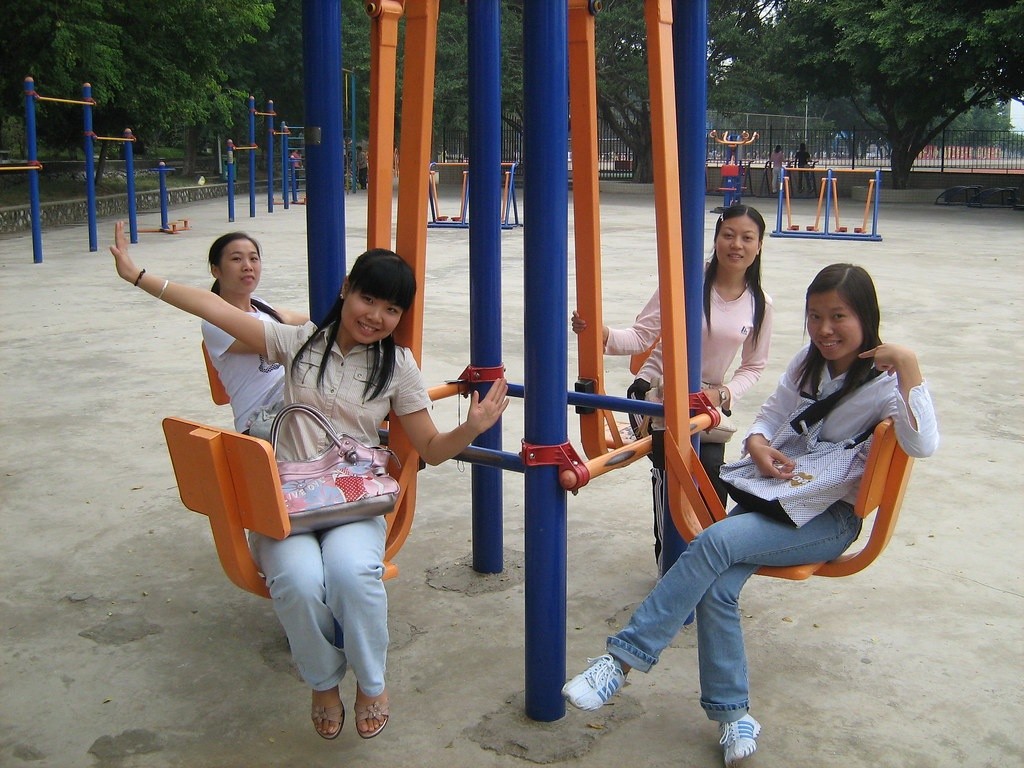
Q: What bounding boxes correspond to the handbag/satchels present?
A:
[634,376,738,443]
[718,366,893,529]
[270,403,401,536]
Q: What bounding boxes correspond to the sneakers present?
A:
[561,653,628,712]
[718,712,763,768]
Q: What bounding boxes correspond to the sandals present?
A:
[354,680,391,739]
[311,684,345,740]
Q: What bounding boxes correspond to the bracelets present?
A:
[134,269,145,286]
[157,280,169,299]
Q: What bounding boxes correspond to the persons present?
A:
[108,222,511,737]
[705,144,841,194]
[559,264,939,768]
[199,231,313,435]
[291,144,367,193]
[571,204,774,626]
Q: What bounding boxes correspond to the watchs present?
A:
[717,388,729,407]
